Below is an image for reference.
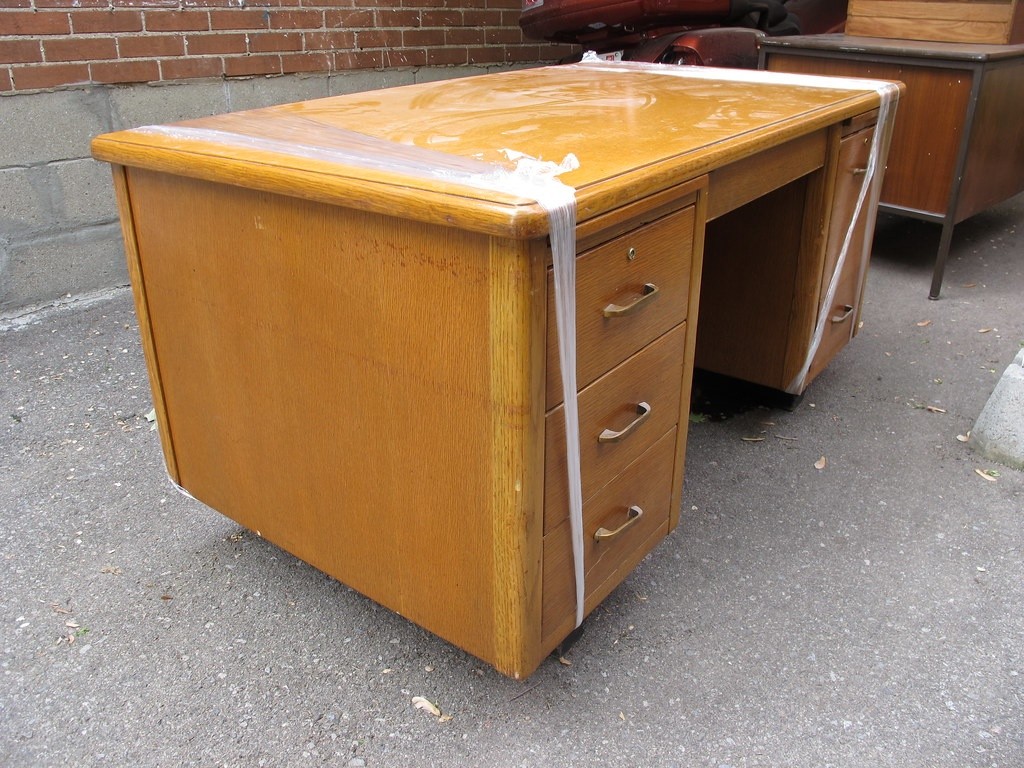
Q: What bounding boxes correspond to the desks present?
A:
[88,59,905,680]
[750,31,1024,300]
